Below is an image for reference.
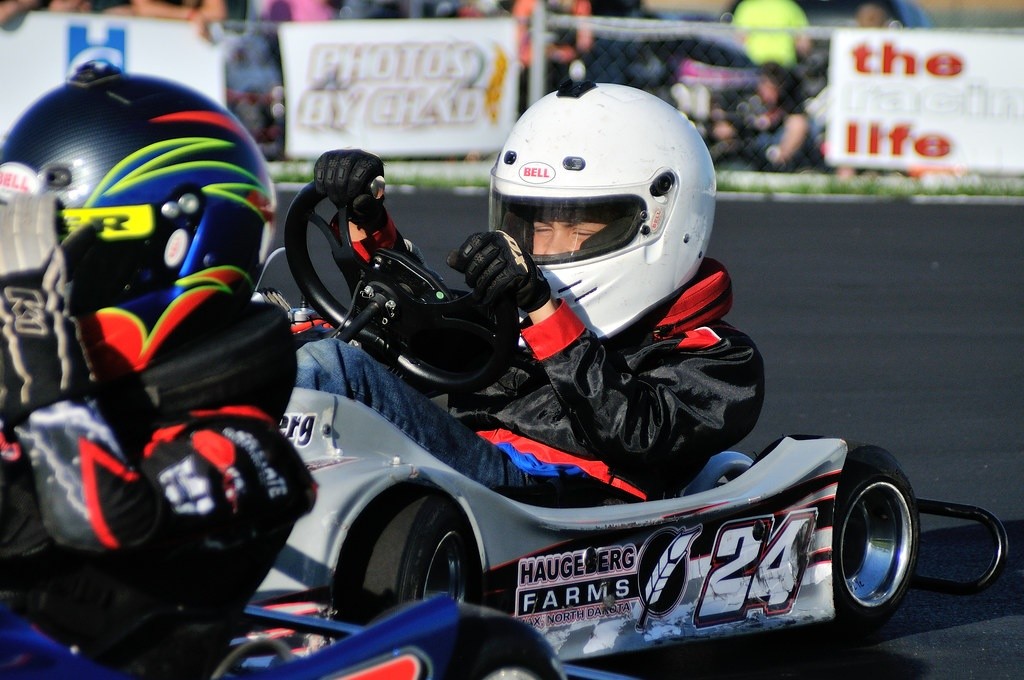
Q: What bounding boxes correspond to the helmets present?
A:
[488,80,718,349]
[1,58,275,379]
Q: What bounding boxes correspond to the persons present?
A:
[287,79,765,508]
[1,71,303,679]
[1,1,903,170]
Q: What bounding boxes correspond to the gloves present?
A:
[446,230,551,314]
[314,149,387,226]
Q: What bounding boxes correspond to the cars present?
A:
[561,8,769,157]
